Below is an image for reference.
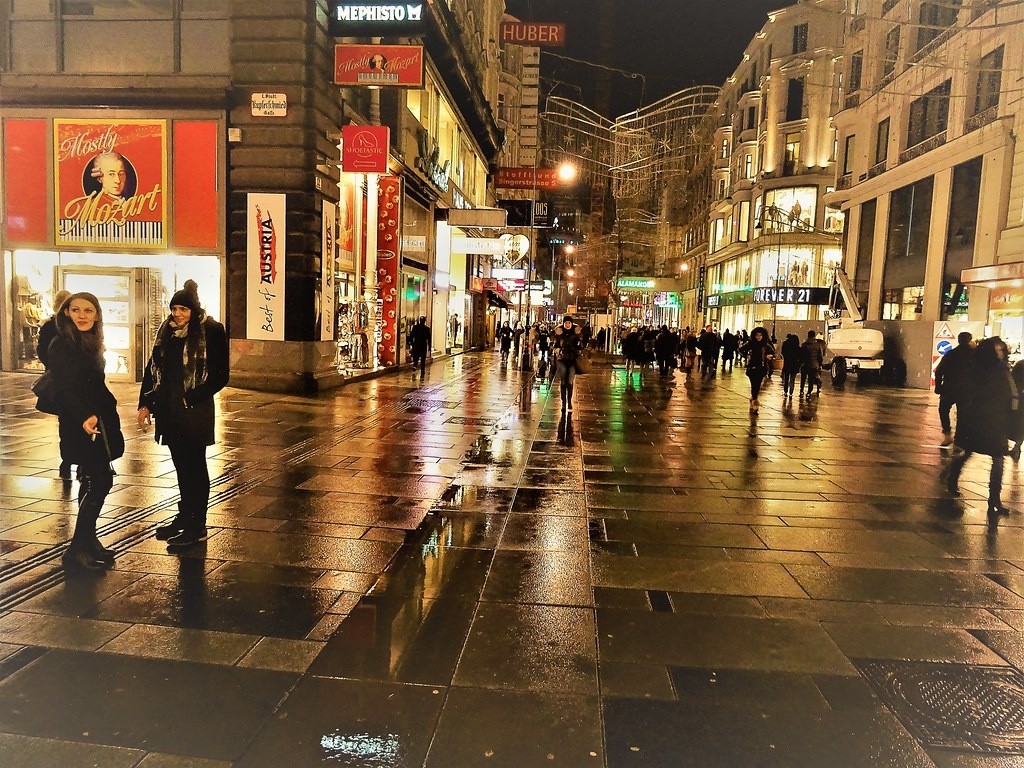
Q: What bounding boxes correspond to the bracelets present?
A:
[143,407,148,410]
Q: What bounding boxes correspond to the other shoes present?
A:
[942,435,953,446]
[60,461,72,471]
[1008,446,1021,454]
[76,465,82,477]
[750,399,761,407]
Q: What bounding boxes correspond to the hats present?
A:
[170,279,201,314]
[563,316,573,324]
[53,290,71,313]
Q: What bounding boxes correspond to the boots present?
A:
[569,399,572,409]
[157,501,185,537]
[167,510,207,546]
[562,401,566,412]
[62,491,105,572]
[78,482,115,560]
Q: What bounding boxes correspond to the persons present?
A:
[410,316,432,381]
[450,314,460,347]
[581,323,592,348]
[941,336,1024,515]
[36,291,125,572]
[597,327,605,350]
[496,321,500,341]
[501,321,525,363]
[138,280,229,546]
[724,327,776,413]
[554,316,581,413]
[614,324,723,379]
[935,332,973,451]
[526,322,558,361]
[780,331,823,401]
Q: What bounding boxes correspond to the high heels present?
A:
[988,491,1011,513]
[947,475,960,496]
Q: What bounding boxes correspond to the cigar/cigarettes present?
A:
[137,428,148,431]
[92,428,97,441]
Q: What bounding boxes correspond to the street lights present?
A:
[521,143,576,369]
[549,235,575,321]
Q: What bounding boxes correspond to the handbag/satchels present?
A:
[576,350,591,374]
[32,369,50,396]
[769,352,784,370]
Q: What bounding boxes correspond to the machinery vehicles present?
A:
[816,261,885,386]
